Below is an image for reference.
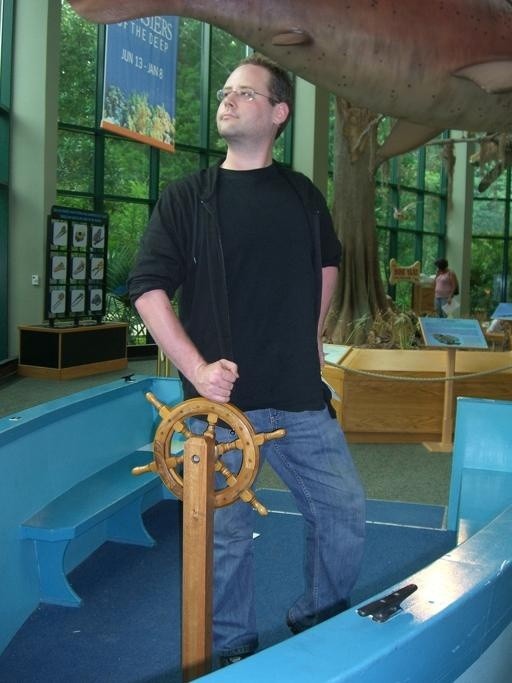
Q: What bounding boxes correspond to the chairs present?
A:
[219,648,254,667]
[284,607,333,634]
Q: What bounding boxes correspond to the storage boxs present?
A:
[17,437,191,609]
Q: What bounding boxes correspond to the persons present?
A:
[432,259,459,316]
[125,57,368,669]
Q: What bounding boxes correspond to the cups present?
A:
[215,85,280,103]
